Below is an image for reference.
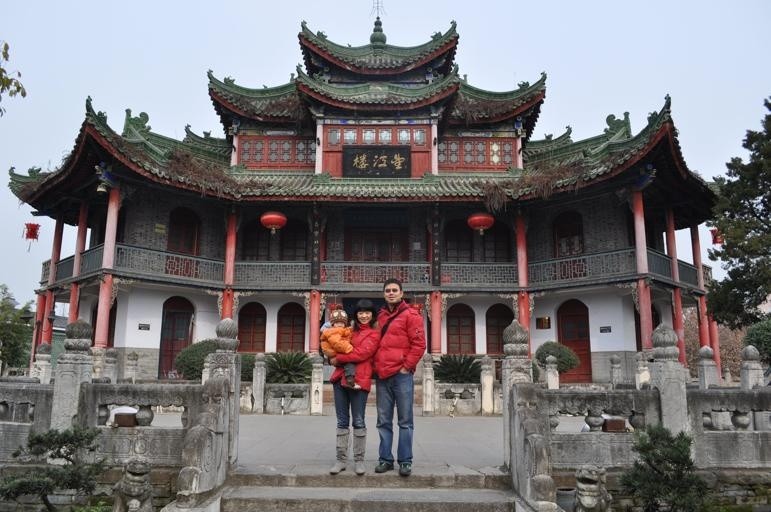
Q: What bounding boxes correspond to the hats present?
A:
[330,304,348,326]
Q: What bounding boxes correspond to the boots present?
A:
[330,428,349,474]
[354,428,366,475]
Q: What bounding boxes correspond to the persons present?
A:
[328,298,381,477]
[317,303,361,392]
[371,278,426,477]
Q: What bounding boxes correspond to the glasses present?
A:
[386,288,398,292]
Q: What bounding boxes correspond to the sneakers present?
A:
[375,463,393,473]
[399,463,411,475]
[349,383,361,390]
